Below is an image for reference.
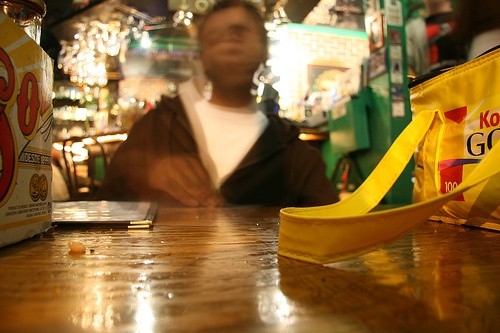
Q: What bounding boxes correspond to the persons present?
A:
[92,0,341,207]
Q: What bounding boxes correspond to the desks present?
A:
[1,203,500,332]
